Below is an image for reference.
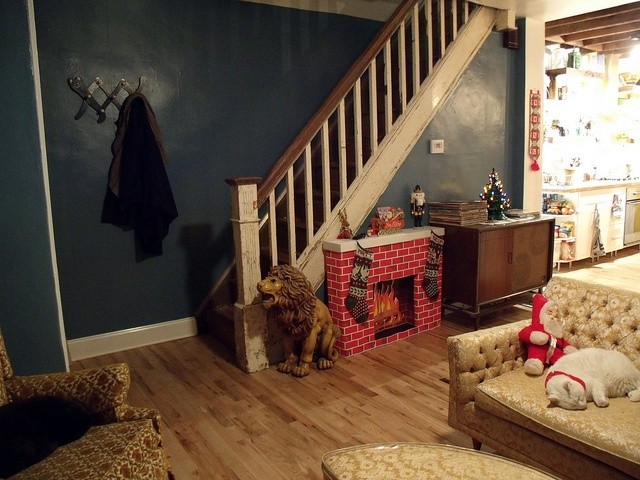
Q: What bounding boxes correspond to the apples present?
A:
[561,208,568,215]
[567,208,574,215]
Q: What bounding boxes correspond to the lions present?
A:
[256,264,342,378]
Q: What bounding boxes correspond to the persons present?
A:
[519,293,578,377]
[409,184,426,228]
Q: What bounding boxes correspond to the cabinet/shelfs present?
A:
[624,184,640,250]
[542,183,626,272]
[428,213,555,332]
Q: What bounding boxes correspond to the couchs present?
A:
[0,334,176,480]
[446,276,640,480]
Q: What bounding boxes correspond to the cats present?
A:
[544,347,640,411]
